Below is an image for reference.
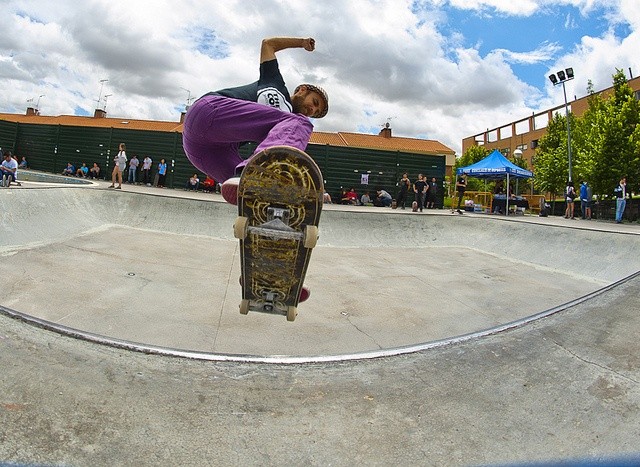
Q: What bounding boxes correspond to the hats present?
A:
[294,83,327,118]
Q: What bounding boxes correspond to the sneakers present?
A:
[239,274,309,303]
[220,175,241,206]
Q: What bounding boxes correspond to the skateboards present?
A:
[392,198,397,209]
[15,179,24,185]
[412,201,417,211]
[233,145,324,321]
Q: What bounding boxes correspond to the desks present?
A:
[492,200,529,215]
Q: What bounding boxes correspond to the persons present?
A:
[360,191,371,205]
[613,176,627,224]
[396,173,411,209]
[108,142,128,190]
[579,181,587,219]
[491,182,501,213]
[76,162,88,178]
[428,177,438,209]
[584,182,594,220]
[456,174,468,214]
[201,174,215,193]
[564,181,577,219]
[182,36,330,205]
[141,155,153,186]
[157,158,168,188]
[423,175,427,209]
[499,180,506,195]
[62,162,75,175]
[377,189,392,207]
[12,154,18,160]
[128,155,139,185]
[89,161,101,179]
[335,188,357,205]
[324,189,332,205]
[412,173,428,211]
[0,151,18,184]
[346,189,357,203]
[184,172,200,192]
[506,183,513,197]
[18,156,27,168]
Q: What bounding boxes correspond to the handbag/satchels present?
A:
[566,189,576,200]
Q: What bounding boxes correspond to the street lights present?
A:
[548,68,574,217]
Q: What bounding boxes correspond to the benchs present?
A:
[523,195,547,216]
[452,191,492,214]
